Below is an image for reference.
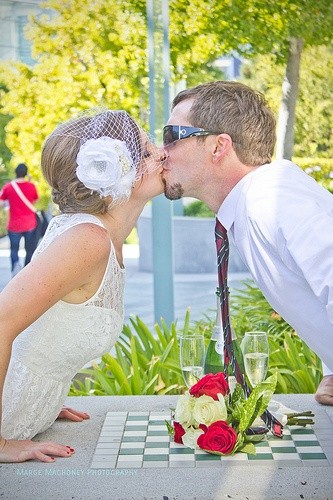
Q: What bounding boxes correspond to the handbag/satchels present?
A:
[35,210,49,238]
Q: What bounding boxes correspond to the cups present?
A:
[180,335,205,389]
[243,332,269,389]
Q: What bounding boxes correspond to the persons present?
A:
[0,164,41,274]
[163,80,333,440]
[0,110,166,462]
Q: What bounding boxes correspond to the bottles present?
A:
[205,287,245,397]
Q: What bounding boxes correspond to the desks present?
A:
[0,392,333,500]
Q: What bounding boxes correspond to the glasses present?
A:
[162,125,240,150]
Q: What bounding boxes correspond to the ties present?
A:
[214,217,284,440]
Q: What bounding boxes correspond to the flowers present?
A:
[75,136,136,199]
[163,371,316,458]
